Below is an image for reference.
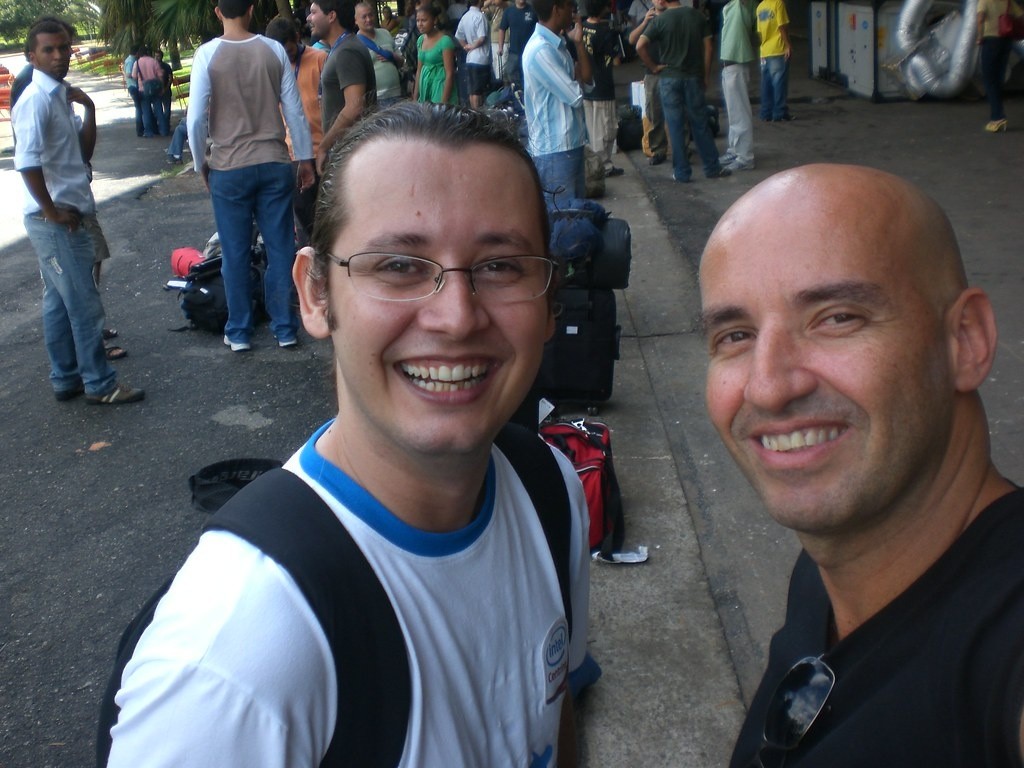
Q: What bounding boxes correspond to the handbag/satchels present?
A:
[142,77,163,96]
[535,421,625,564]
[999,0,1024,39]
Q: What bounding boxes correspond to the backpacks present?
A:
[167,255,264,331]
[171,231,264,276]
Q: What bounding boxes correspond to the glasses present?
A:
[321,251,560,303]
[747,652,836,768]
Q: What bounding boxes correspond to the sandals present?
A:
[104,346,127,358]
[104,327,118,338]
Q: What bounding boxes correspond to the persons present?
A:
[11,15,127,358]
[699,163,1024,768]
[10,20,145,404]
[186,0,315,351]
[974,0,1024,132]
[756,0,791,119]
[104,99,602,768]
[523,0,591,274]
[260,0,759,181]
[126,45,173,136]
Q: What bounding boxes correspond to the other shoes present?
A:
[650,154,666,166]
[224,335,251,351]
[273,335,298,347]
[604,166,624,177]
[711,169,732,178]
[773,115,796,121]
[763,115,773,121]
[169,154,182,164]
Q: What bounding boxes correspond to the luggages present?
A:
[539,184,631,417]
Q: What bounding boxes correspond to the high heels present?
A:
[987,119,1008,133]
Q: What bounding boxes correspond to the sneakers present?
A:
[725,161,754,170]
[719,153,737,164]
[54,381,85,399]
[86,381,147,406]
